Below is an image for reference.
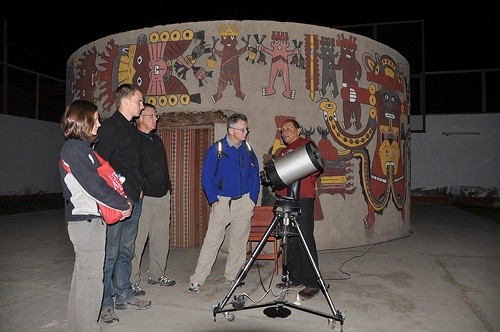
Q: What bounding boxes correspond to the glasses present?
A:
[230,127,249,132]
[141,114,159,119]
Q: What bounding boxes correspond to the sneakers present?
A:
[132,284,145,296]
[115,298,152,310]
[188,283,200,293]
[225,279,242,287]
[148,276,176,286]
[100,307,119,326]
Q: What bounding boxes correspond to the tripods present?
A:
[212,196,344,326]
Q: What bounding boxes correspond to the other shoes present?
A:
[276,283,302,289]
[298,287,319,298]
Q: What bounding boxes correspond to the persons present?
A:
[129,103,175,295]
[60,100,133,332]
[188,113,260,292]
[96,83,152,324]
[275,119,320,299]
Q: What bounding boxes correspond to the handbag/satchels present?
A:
[60,150,129,225]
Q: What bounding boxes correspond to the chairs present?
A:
[247,206,279,274]
